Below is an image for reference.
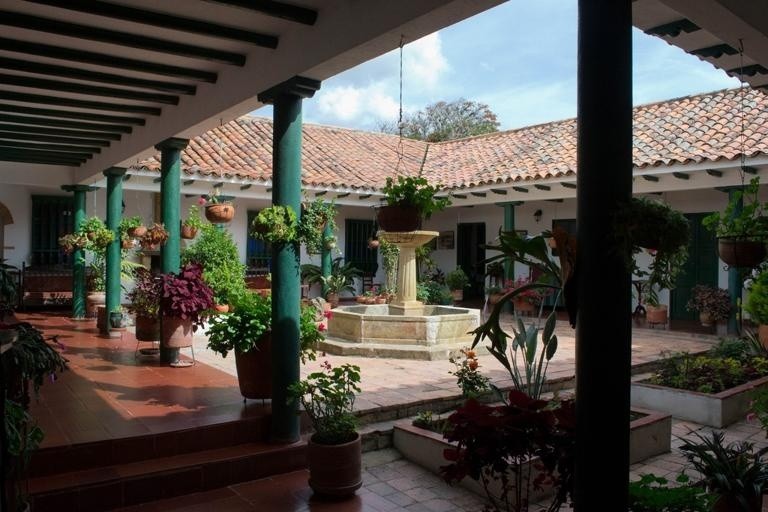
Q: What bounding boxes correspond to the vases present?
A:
[512,299,536,312]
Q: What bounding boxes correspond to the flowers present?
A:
[503,276,555,304]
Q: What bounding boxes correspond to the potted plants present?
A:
[701,176,768,268]
[321,274,346,306]
[156,261,214,367]
[57,184,395,262]
[446,264,472,302]
[646,288,668,324]
[120,266,163,364]
[357,286,392,306]
[284,361,364,497]
[84,262,106,319]
[205,263,247,313]
[737,261,768,355]
[683,283,732,328]
[371,174,454,232]
[205,291,326,407]
[484,283,504,305]
